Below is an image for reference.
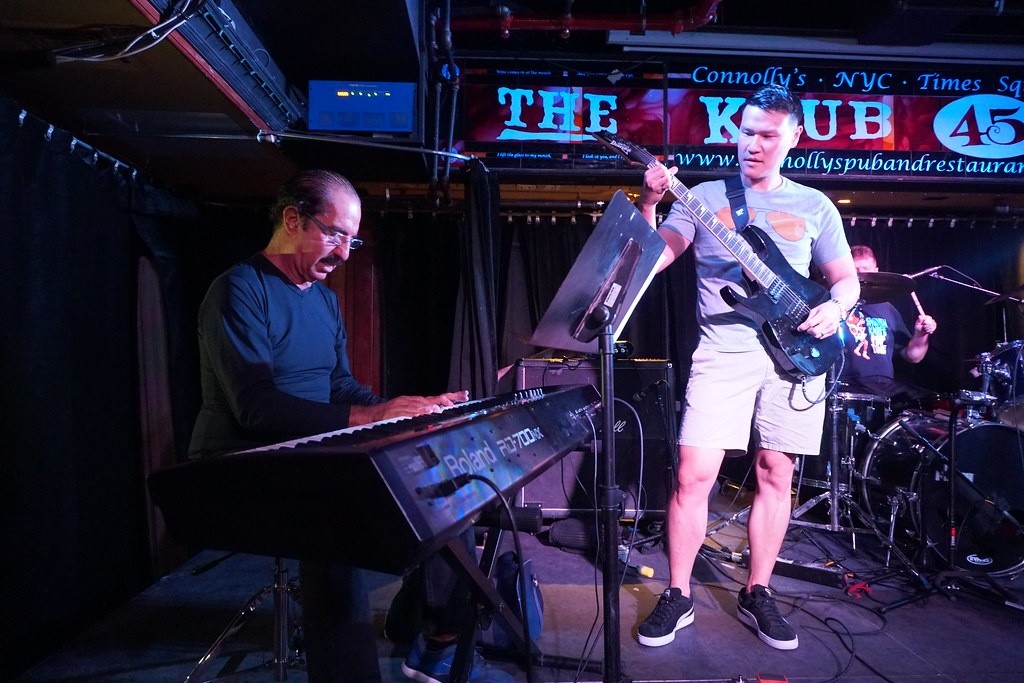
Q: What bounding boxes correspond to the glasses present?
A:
[301,210,360,250]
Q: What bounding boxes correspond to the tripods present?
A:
[789,358,1024,619]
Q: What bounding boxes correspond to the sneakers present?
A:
[737,584,799,650]
[638,588,695,647]
[401,633,518,683]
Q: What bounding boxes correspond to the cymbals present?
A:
[984,282,1024,307]
[938,360,980,393]
[859,273,919,306]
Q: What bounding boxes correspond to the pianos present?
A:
[147,382,607,575]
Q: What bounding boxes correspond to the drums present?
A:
[959,339,1024,431]
[791,391,893,488]
[860,410,1024,578]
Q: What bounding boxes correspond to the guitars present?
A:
[591,129,848,382]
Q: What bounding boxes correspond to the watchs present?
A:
[832,298,847,322]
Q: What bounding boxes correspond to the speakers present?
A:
[494,358,677,526]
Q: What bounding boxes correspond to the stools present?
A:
[142,466,303,683]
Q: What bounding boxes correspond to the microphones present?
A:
[902,265,942,278]
[632,379,662,401]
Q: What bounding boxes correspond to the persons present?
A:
[187,169,517,683]
[635,83,861,649]
[838,245,937,466]
